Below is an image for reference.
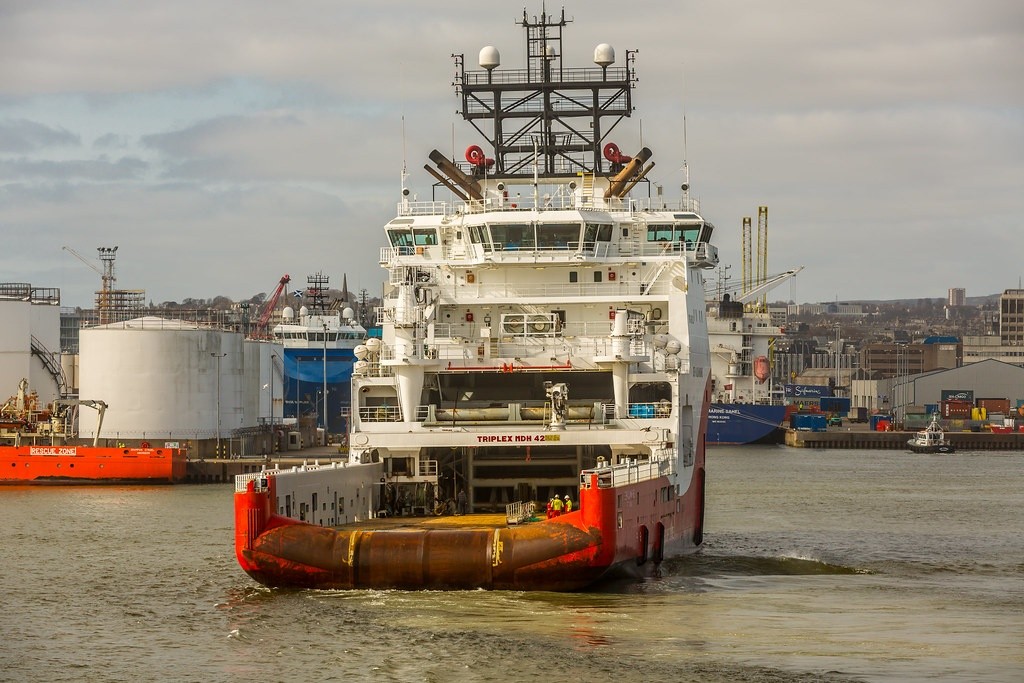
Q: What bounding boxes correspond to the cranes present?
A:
[245,274,292,341]
[60,245,120,282]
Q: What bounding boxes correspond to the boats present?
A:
[907,412,955,453]
[0,378,189,485]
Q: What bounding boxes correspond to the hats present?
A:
[563,494,569,498]
[554,494,559,498]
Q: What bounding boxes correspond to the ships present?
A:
[230,1,721,596]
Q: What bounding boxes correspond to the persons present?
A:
[550,494,565,518]
[458,489,468,515]
[546,498,556,519]
[564,495,572,514]
